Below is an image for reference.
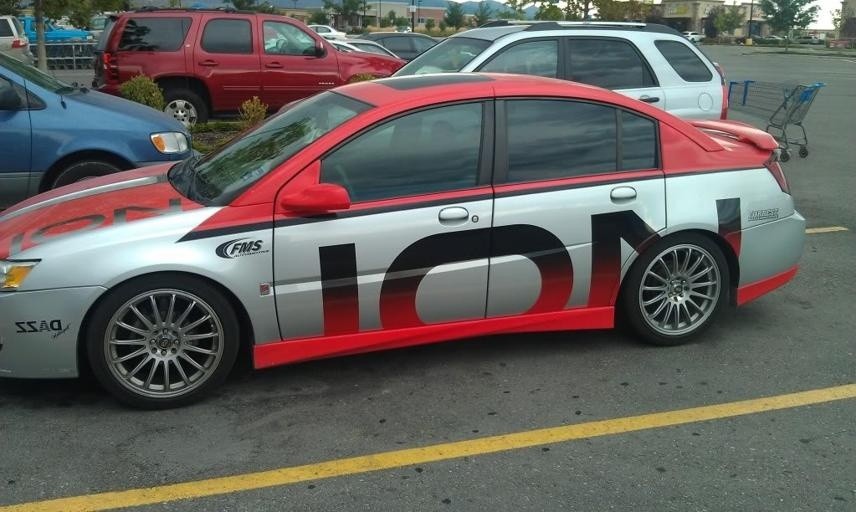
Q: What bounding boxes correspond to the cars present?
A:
[91,8,410,131]
[764,35,785,45]
[392,17,731,125]
[22,16,96,47]
[0,12,35,70]
[682,30,706,43]
[264,25,438,62]
[0,72,809,414]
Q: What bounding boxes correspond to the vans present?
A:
[798,36,818,44]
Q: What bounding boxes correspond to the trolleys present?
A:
[728,77,825,161]
